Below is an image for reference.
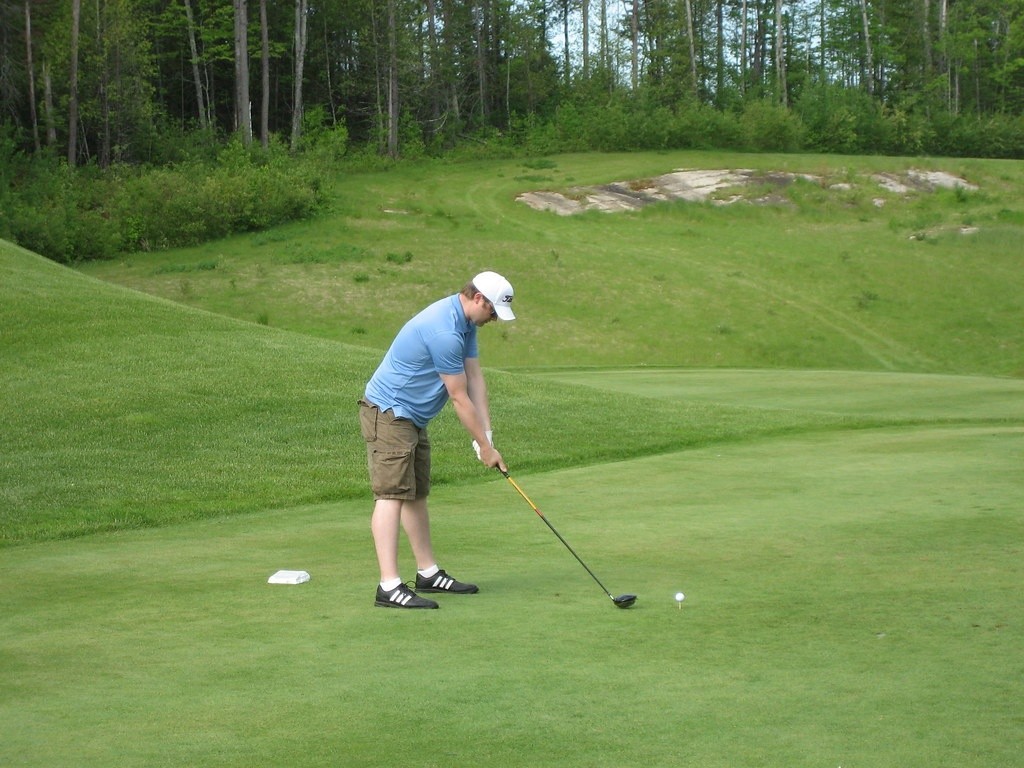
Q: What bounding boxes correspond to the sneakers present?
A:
[415,570,479,594]
[374,583,439,609]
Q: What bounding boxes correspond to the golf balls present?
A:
[676,593,684,601]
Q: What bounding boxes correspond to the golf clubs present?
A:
[495,462,638,609]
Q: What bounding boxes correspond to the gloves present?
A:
[472,431,494,461]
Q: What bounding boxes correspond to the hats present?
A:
[472,270,516,320]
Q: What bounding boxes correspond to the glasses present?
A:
[487,301,496,317]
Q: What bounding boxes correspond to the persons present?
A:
[356,271,516,610]
